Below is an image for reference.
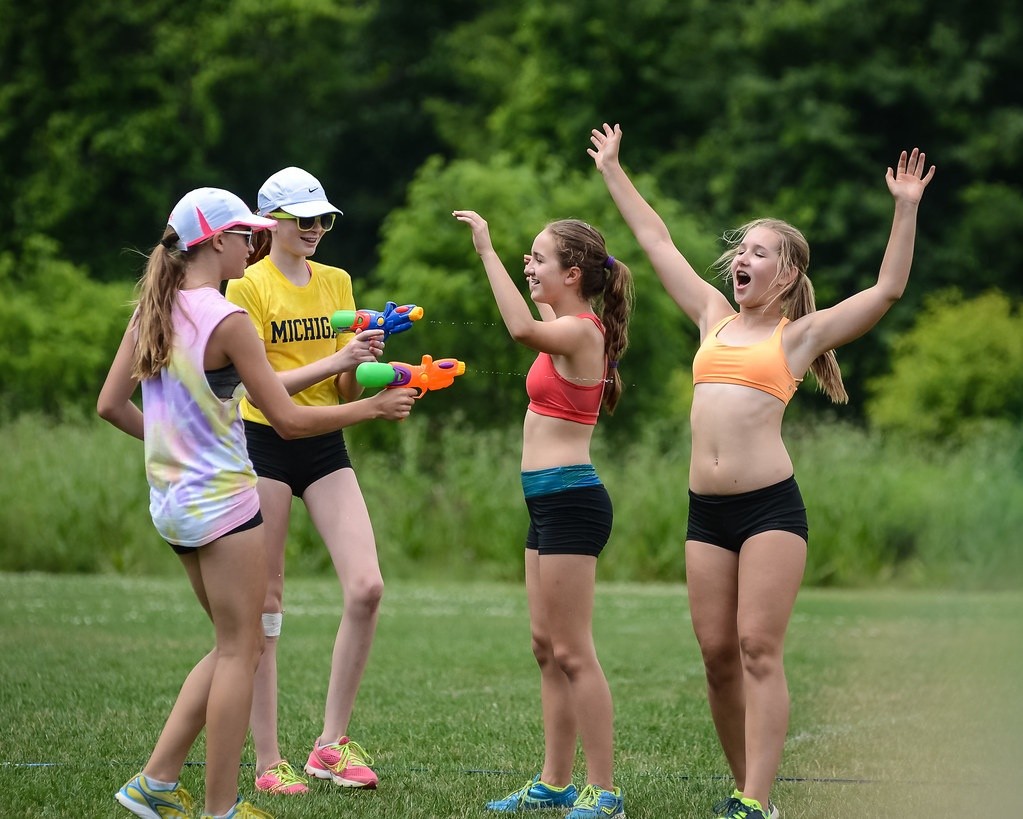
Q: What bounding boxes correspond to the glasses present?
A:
[270,212,336,232]
[200,229,252,245]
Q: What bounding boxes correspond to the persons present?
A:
[585,121,936,818]
[452,211,636,818]
[96,186,420,819]
[224,167,384,798]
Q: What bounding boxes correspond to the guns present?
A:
[331,302,426,345]
[353,353,467,398]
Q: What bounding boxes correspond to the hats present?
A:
[167,187,277,252]
[257,167,343,218]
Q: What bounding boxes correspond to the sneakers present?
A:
[565,784,626,819]
[485,771,577,812]
[255,760,311,796]
[304,735,378,789]
[114,767,194,819]
[200,796,275,819]
[712,787,779,819]
